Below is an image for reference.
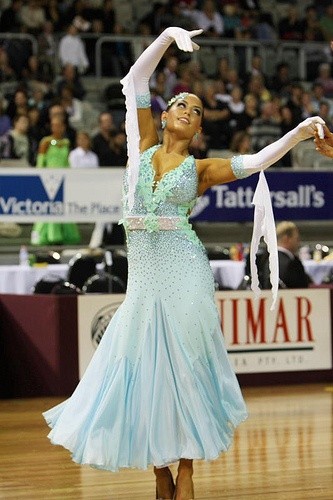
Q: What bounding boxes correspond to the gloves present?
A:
[128,26,203,110]
[230,116,325,180]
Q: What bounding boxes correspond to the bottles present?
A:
[19,245,28,266]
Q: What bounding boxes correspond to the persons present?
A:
[0,0,333,295]
[42,27,326,500]
[311,119,333,157]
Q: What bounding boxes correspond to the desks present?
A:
[0,258,333,395]
